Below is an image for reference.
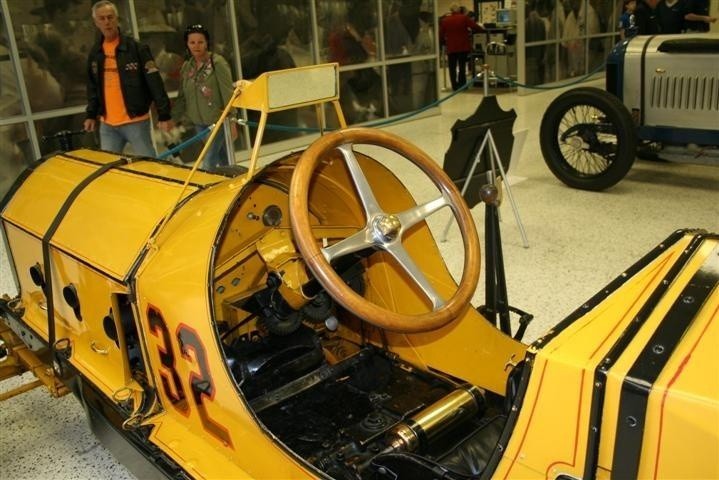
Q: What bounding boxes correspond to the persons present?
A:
[618,0,719,44]
[162,25,238,171]
[84,2,174,160]
[437,4,487,92]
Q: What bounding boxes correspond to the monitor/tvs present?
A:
[496,8,516,27]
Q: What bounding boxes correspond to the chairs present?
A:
[370,357,526,479]
